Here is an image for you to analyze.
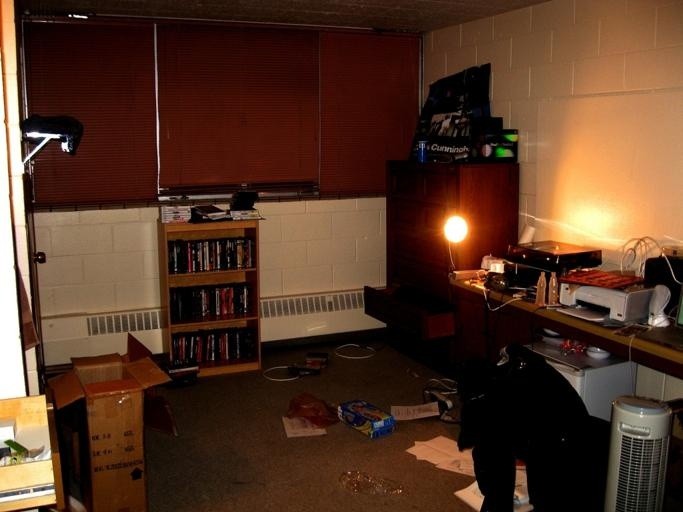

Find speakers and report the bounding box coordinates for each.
[650,286,672,328]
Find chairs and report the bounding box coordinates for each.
[468,345,613,511]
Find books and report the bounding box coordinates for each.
[160,199,259,386]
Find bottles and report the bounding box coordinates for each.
[548,272,556,305]
[535,271,546,305]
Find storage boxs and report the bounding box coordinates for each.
[44,331,170,512]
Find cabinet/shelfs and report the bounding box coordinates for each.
[156,216,262,378]
[383,159,519,364]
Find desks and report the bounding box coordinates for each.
[448,267,683,381]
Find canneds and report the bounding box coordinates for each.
[418,140,428,163]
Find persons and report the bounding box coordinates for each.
[426,111,469,138]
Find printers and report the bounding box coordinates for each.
[557,281,650,324]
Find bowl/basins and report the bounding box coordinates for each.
[585,348,609,359]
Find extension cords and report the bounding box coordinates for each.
[427,390,455,410]
